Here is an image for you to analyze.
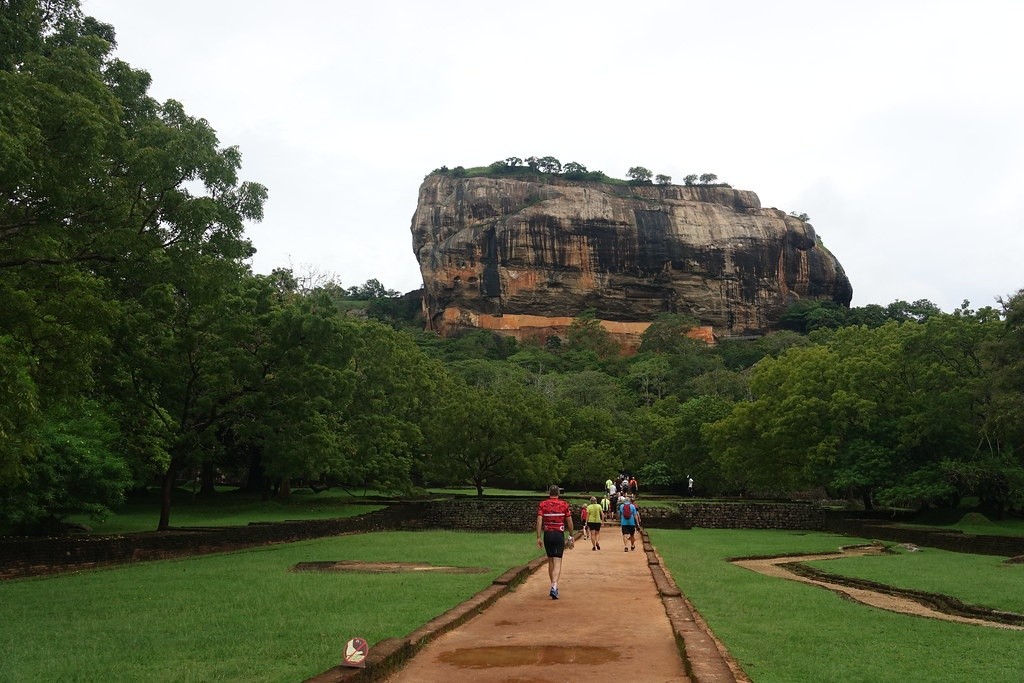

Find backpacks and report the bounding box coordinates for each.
[623,503,632,519]
[631,480,636,491]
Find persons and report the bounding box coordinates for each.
[535,474,694,601]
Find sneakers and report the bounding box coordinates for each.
[550,589,559,599]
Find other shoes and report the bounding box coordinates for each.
[624,546,628,551]
[584,536,589,540]
[592,543,600,551]
[631,546,635,550]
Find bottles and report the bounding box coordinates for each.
[568,535,572,550]
